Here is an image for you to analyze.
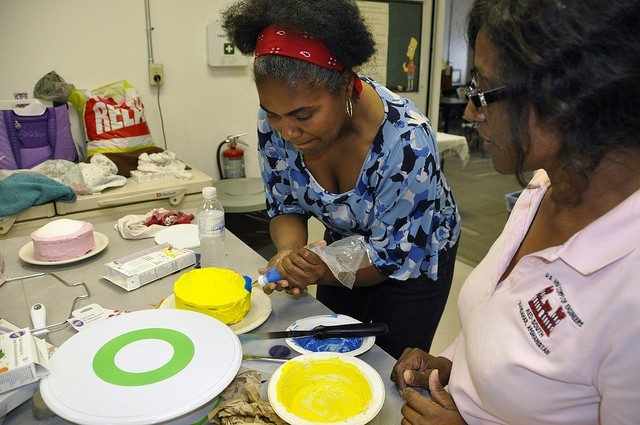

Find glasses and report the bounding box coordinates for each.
[465,69,530,109]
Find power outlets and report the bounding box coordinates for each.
[148,62,163,87]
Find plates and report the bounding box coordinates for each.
[154,224,203,248]
[268,352,385,425]
[18,231,109,266]
[159,285,273,334]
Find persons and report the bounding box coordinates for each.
[219,1,463,361]
[390,0,640,425]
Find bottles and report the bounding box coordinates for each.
[198,186,227,268]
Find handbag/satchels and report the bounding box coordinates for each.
[70,79,155,175]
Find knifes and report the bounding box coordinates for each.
[239,324,386,340]
[286,314,377,357]
[242,355,292,362]
[31,304,53,346]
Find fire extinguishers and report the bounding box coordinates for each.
[217,132,249,180]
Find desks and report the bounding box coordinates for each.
[432,129,469,173]
[440,97,469,133]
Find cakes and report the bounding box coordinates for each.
[27,213,98,259]
[175,264,254,324]
